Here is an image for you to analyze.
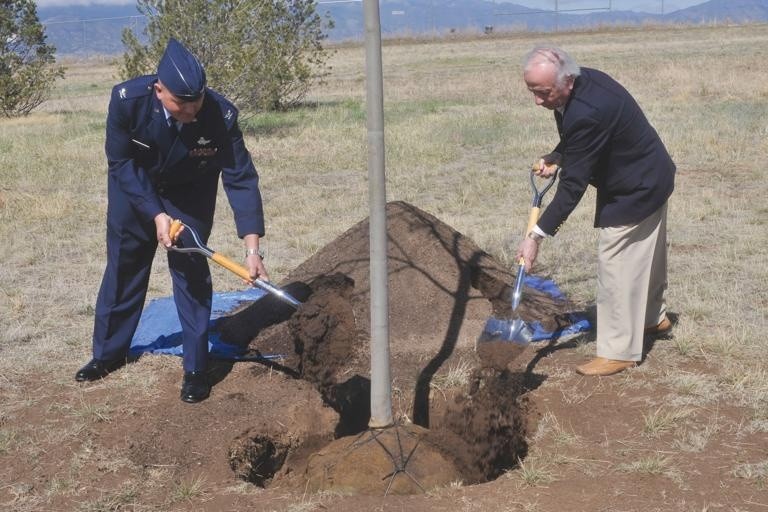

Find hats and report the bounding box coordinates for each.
[156,35,207,103]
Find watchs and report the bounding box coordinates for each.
[528,232,543,245]
[244,248,265,261]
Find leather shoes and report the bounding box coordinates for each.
[179,370,212,404]
[646,315,673,337]
[576,356,636,377]
[74,357,124,382]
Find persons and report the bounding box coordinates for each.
[75,37,270,403]
[516,44,676,377]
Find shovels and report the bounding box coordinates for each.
[476,162,558,365]
[164,219,357,352]
